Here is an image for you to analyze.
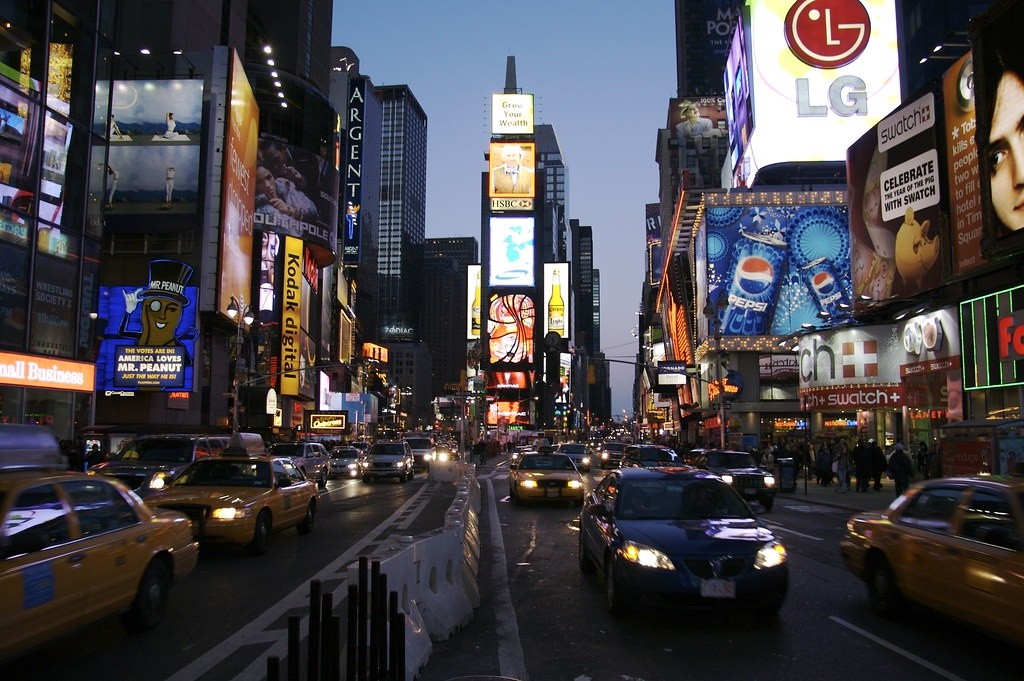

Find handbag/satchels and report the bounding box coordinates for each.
[831,462,838,473]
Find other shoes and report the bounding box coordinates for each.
[842,490,847,494]
[855,488,860,492]
[861,489,868,493]
[834,486,838,492]
[817,481,819,484]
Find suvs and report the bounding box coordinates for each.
[362,439,415,483]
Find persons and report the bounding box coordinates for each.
[254,136,320,237]
[504,438,527,454]
[468,438,487,466]
[57,437,129,468]
[96,102,184,208]
[317,438,354,451]
[676,99,712,139]
[654,439,742,458]
[492,145,534,194]
[672,481,723,521]
[972,30,1024,263]
[760,432,941,496]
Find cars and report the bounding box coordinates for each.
[683,448,775,509]
[328,446,362,479]
[579,467,789,624]
[85,435,231,499]
[142,433,320,557]
[403,431,435,470]
[618,444,682,470]
[266,442,331,488]
[555,443,593,472]
[509,438,585,507]
[0,423,198,658]
[601,442,626,469]
[841,476,1024,645]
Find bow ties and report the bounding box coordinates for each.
[505,169,518,175]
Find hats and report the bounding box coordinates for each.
[679,99,698,119]
[868,439,874,443]
[895,443,903,450]
